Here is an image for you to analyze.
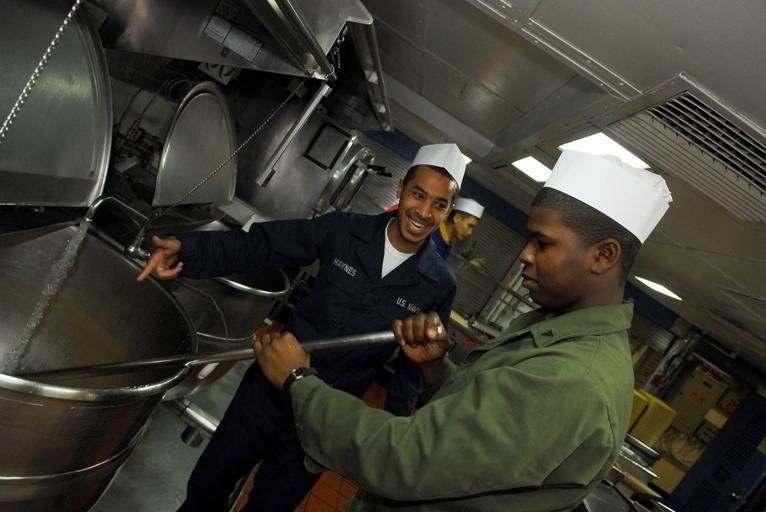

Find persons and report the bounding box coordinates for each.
[133,164,458,510]
[433,208,480,266]
[253,187,643,510]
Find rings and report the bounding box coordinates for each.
[156,266,167,271]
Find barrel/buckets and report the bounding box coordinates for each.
[151,79,291,392]
[0,1,200,512]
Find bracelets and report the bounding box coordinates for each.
[279,366,316,391]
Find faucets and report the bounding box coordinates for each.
[83,195,152,263]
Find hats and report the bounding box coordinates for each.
[543,150,674,245]
[409,143,466,191]
[452,198,485,219]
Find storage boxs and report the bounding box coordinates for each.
[627,387,675,449]
